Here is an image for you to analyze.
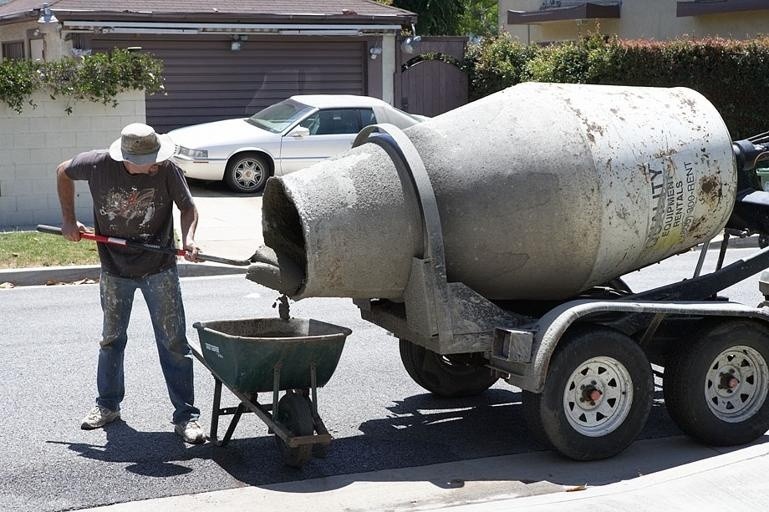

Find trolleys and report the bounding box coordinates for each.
[186,317,352,468]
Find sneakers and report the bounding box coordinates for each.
[173,418,206,444]
[80,401,121,429]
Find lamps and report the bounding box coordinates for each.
[38,6,59,25]
[401,24,421,55]
[370,37,381,59]
[230,34,247,50]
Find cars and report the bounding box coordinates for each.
[165,93,429,193]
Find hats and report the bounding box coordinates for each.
[108,121,175,164]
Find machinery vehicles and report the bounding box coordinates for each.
[263,81,769,461]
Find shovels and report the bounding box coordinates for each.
[36,224,305,297]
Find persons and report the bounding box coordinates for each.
[53,122,211,444]
